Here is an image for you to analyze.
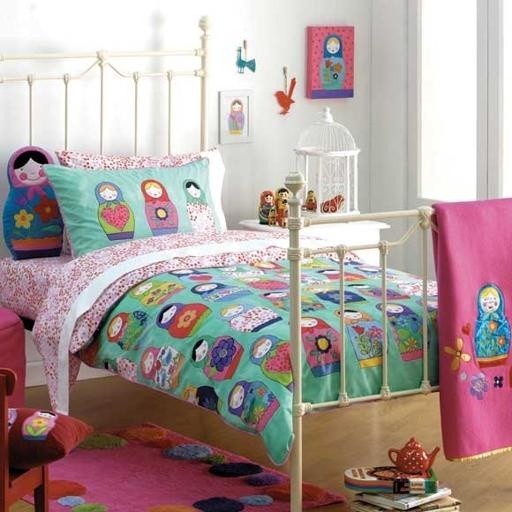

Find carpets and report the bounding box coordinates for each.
[21,420,347,512]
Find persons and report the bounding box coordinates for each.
[307,190,317,211]
[259,186,290,228]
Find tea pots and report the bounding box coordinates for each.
[387,436,441,475]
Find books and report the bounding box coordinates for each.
[350,485,460,511]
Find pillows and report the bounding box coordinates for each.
[42,145,226,261]
[8,408,94,470]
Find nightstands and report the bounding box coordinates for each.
[238,218,391,268]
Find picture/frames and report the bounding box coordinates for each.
[218,90,253,145]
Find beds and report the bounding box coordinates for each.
[0,16,512,511]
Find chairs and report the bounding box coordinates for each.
[0,367,50,512]
